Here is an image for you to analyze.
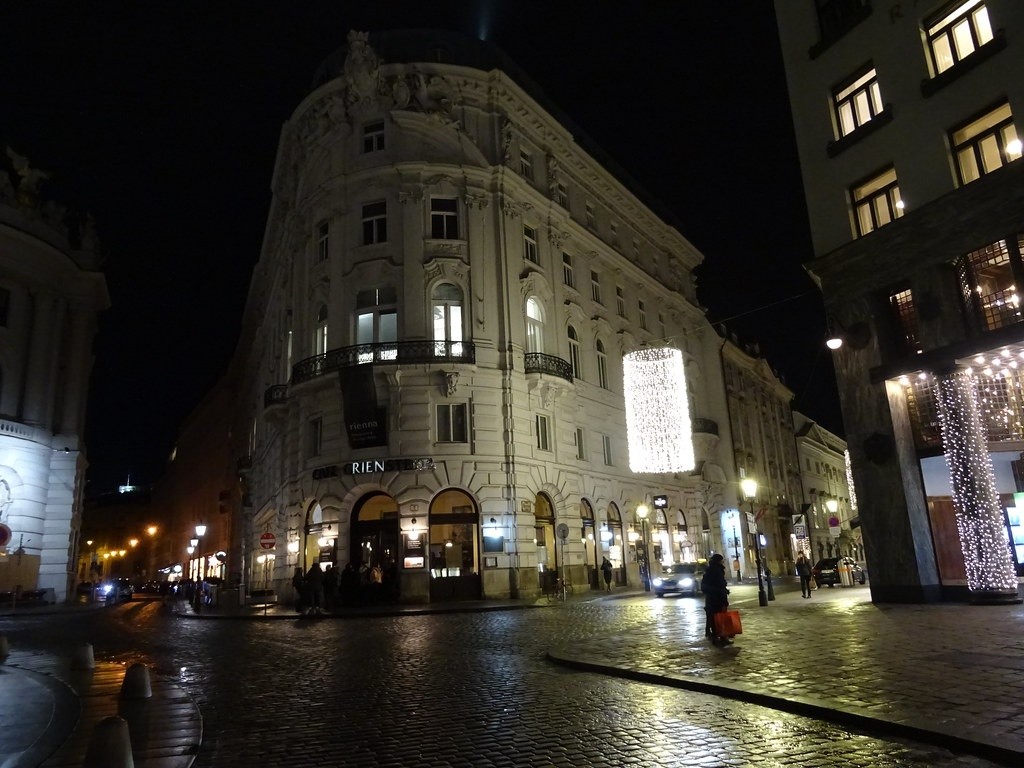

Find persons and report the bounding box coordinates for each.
[170,576,210,614]
[601,559,613,592]
[292,558,383,616]
[344,30,386,104]
[795,550,813,598]
[0,156,99,270]
[702,554,735,647]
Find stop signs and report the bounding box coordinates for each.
[260,533,276,549]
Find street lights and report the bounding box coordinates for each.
[743,479,768,607]
[188,535,202,612]
[826,496,848,588]
[194,519,206,612]
[637,503,651,591]
[824,313,871,350]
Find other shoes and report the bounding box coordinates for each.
[712,639,734,644]
[802,595,806,598]
[607,589,611,592]
[806,596,811,599]
[308,606,321,615]
[705,632,715,637]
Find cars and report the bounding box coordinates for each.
[110,578,133,599]
[653,563,706,597]
[812,557,865,585]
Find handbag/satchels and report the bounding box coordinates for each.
[714,611,742,637]
[809,576,818,591]
[607,566,612,571]
